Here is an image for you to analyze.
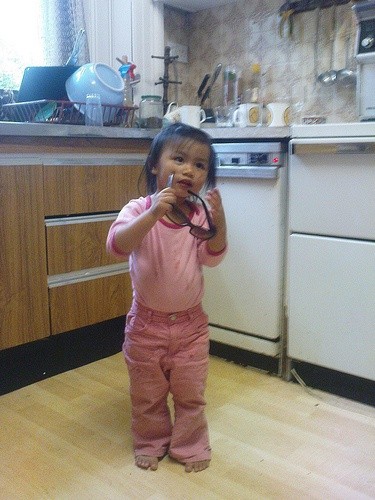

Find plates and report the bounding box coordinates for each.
[34,102,58,123]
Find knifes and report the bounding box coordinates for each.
[192,63,222,108]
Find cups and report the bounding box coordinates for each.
[266,102,290,127]
[181,105,206,129]
[232,103,263,127]
[212,105,236,127]
[85,93,103,126]
[164,101,182,124]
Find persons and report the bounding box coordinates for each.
[105,122,229,472]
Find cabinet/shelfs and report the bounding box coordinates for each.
[0,135,163,396]
[284,137,375,383]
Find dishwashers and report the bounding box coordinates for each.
[187,138,289,378]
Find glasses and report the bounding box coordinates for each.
[165,173,217,240]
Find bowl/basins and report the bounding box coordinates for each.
[16,66,80,100]
[65,63,124,123]
